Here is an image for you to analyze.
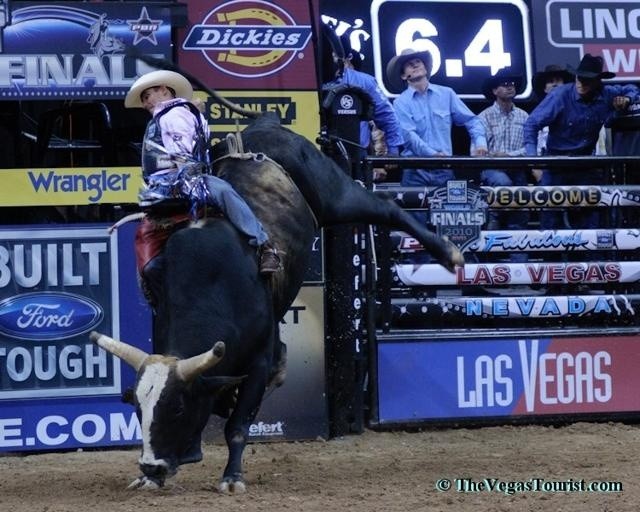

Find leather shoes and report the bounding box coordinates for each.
[260,241,284,273]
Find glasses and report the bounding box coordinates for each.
[500,81,517,86]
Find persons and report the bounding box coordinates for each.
[328,48,640,296]
[124,70,281,303]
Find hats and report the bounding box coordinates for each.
[124,70,193,109]
[386,48,433,90]
[484,53,616,88]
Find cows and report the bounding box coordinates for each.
[87,50,467,495]
